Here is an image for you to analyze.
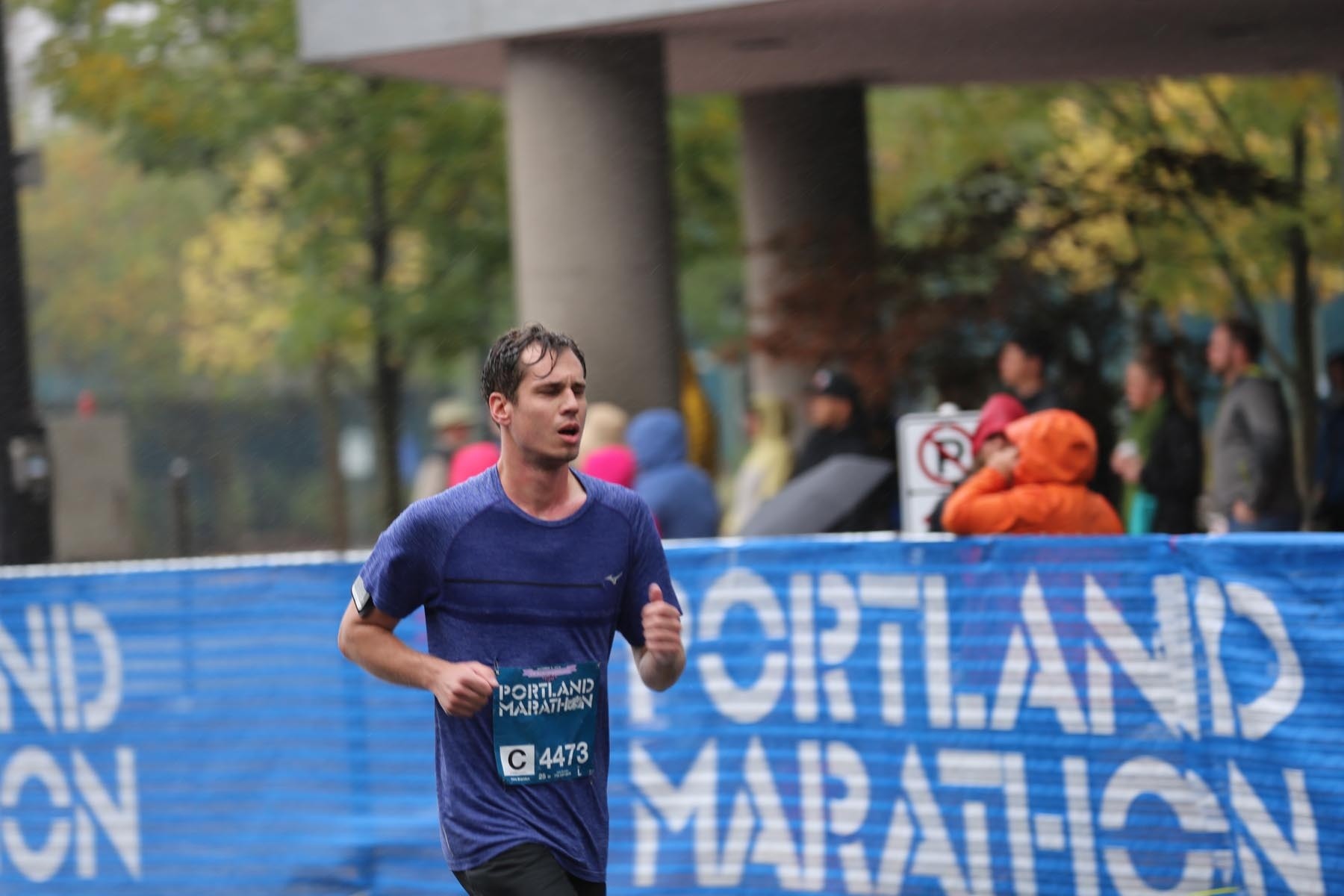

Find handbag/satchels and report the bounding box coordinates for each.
[1126,490,1158,534]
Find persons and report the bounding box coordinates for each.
[335,326,688,896]
[410,323,1344,535]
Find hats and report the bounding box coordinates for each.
[804,368,857,400]
